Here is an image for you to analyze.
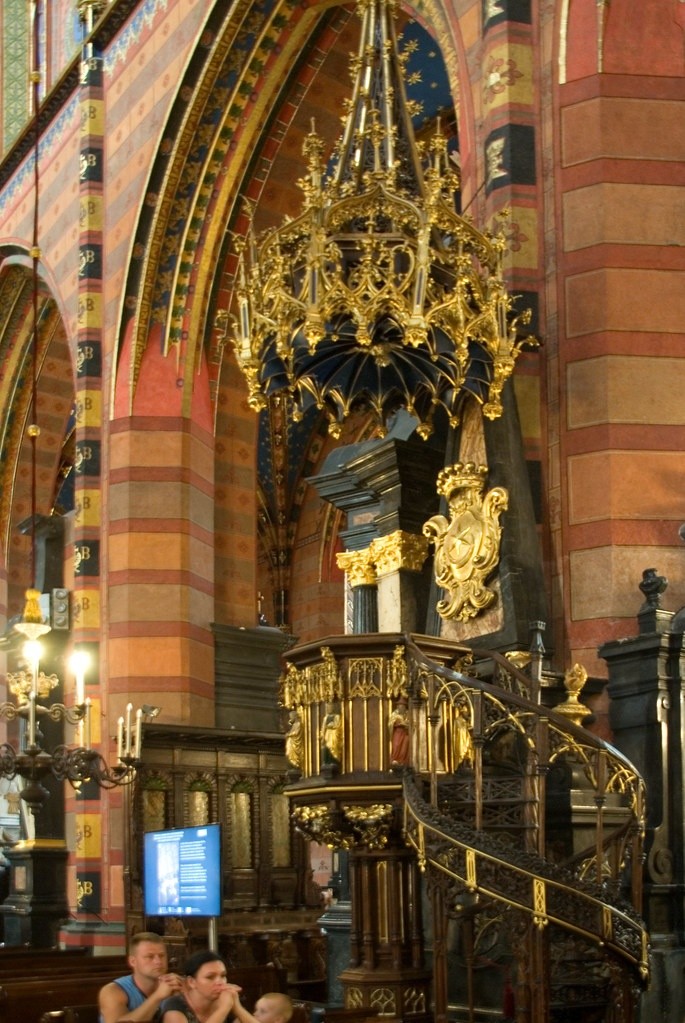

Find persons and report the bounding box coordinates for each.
[159,951,240,1023]
[231,988,292,1022]
[99,932,183,1023]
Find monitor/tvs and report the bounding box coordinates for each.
[143,822,223,917]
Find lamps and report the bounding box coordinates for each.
[0,1,148,816]
